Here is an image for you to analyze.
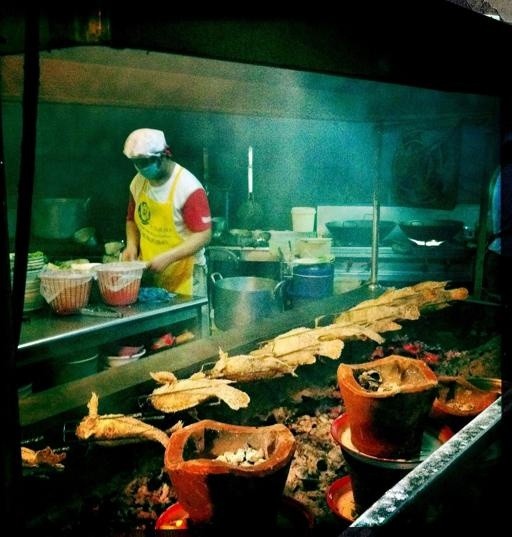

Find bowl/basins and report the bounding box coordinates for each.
[101,345,146,369]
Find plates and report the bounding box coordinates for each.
[10,252,45,318]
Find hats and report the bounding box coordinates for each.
[122,128,169,160]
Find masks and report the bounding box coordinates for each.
[133,162,162,181]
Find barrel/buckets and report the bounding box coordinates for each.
[45,350,98,383]
[103,348,145,372]
[291,207,316,232]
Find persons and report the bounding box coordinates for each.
[120,128,213,340]
[485,138,511,293]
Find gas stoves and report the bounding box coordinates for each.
[330,239,468,265]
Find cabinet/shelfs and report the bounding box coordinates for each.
[16,294,210,388]
[334,244,450,282]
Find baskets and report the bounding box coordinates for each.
[37,269,97,315]
[95,262,146,306]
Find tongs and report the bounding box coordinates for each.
[80,306,123,318]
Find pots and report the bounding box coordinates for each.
[324,213,398,244]
[399,214,464,242]
[30,193,93,240]
[209,272,287,332]
